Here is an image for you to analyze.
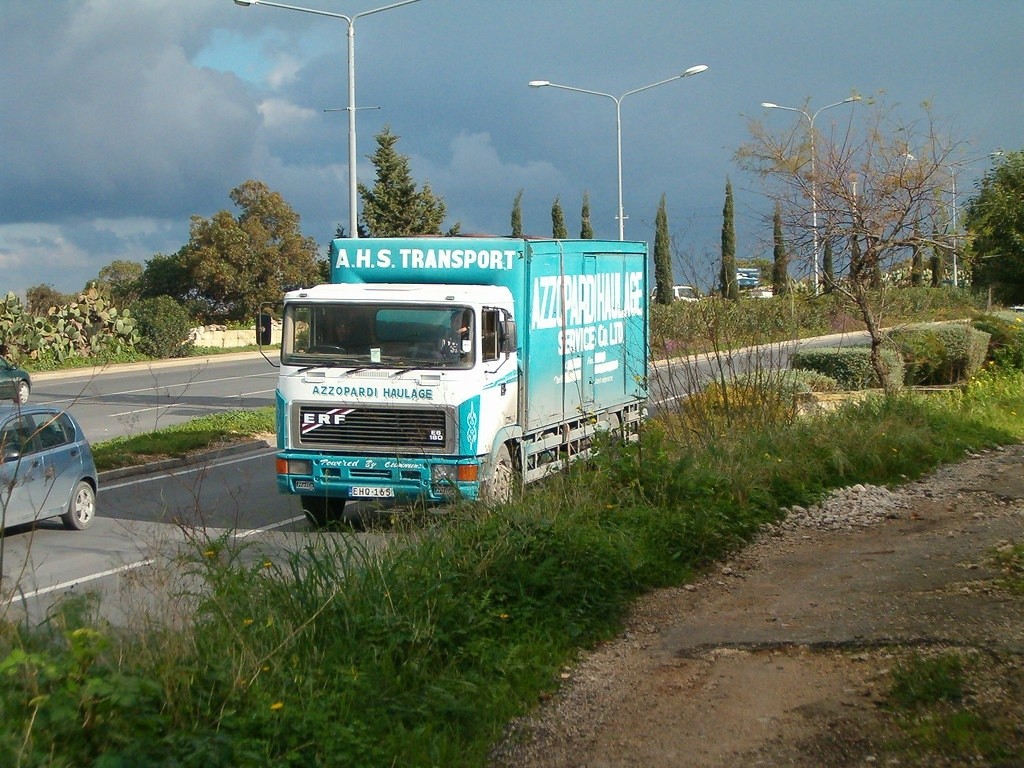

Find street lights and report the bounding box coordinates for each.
[527,64,710,240]
[904,152,1005,287]
[761,94,861,294]
[235,1,423,237]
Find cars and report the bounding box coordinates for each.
[0,407,98,530]
[0,356,32,405]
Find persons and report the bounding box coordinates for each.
[432,312,469,358]
[331,324,367,354]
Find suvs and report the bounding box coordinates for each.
[650,285,703,306]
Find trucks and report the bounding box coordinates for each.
[254,234,650,532]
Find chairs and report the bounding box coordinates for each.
[2,422,59,453]
